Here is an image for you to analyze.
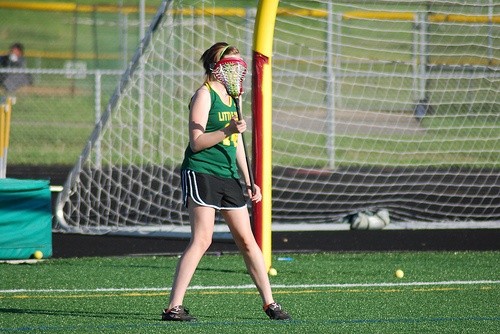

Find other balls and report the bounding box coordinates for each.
[35,251,43,259]
[269,268,277,276]
[395,270,404,279]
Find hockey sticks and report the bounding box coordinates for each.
[209,57,255,196]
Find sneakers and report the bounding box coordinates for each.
[162,305,197,322]
[262,302,291,320]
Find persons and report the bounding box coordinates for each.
[1,43,33,88]
[162,42,291,322]
